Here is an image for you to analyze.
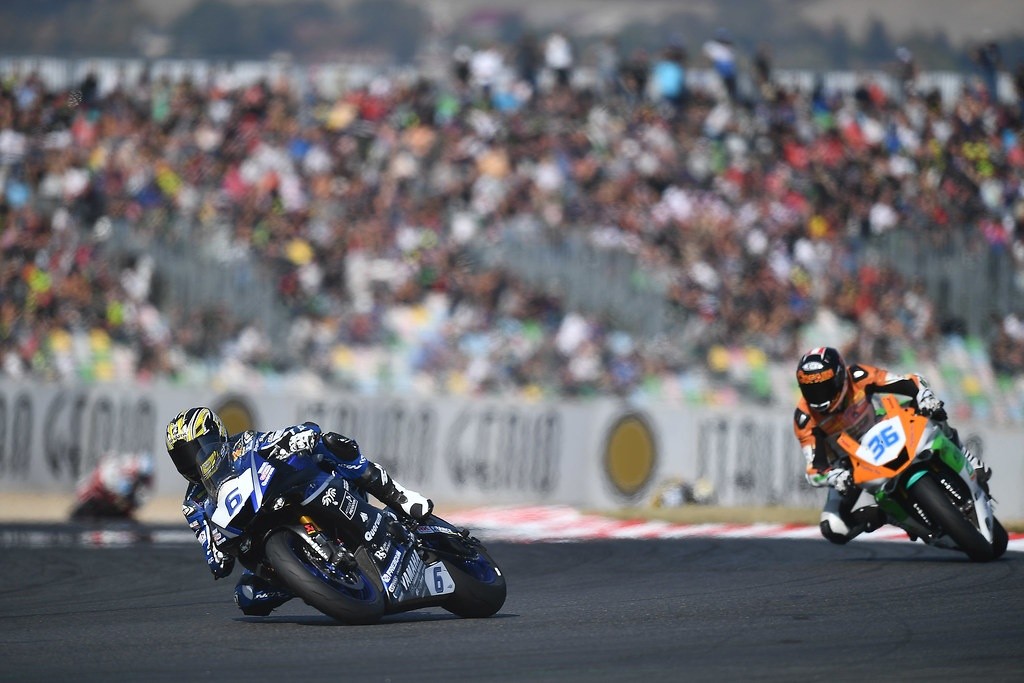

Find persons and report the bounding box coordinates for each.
[95,448,154,514]
[0,32,1024,396]
[165,407,434,616]
[793,347,992,544]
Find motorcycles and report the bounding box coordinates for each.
[208,440,506,626]
[841,394,1009,564]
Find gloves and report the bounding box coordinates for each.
[211,552,235,580]
[917,395,949,421]
[826,467,857,498]
[287,428,320,456]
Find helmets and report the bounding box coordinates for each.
[796,347,849,414]
[164,406,229,483]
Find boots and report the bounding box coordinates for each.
[361,461,434,523]
[946,426,992,484]
